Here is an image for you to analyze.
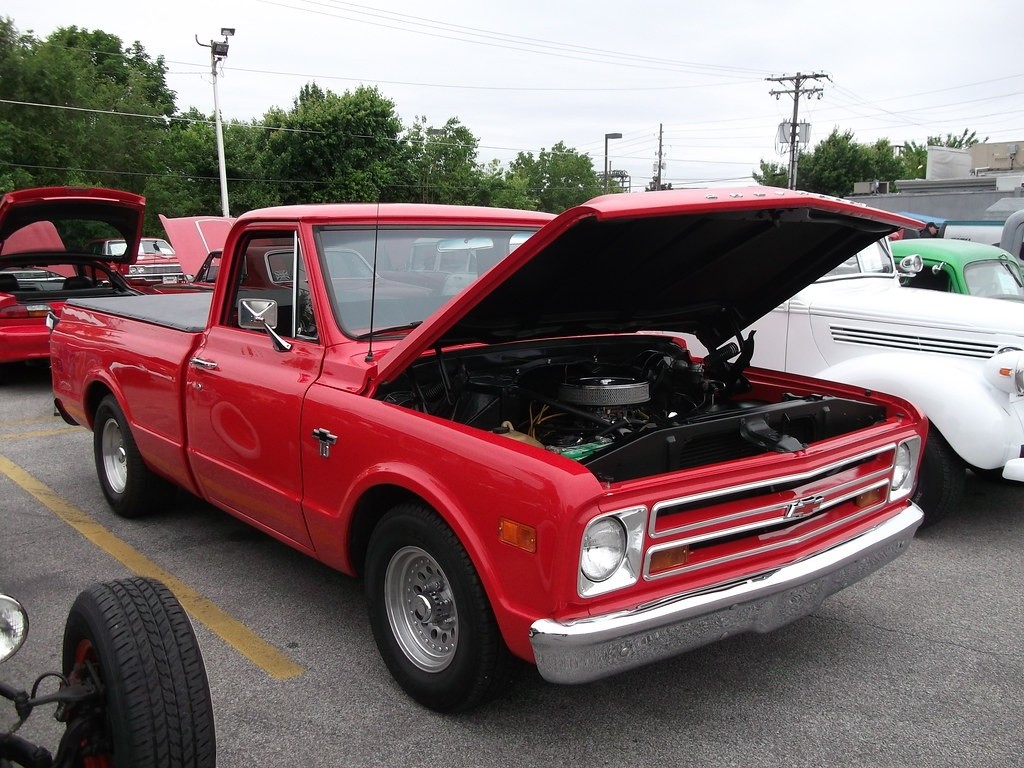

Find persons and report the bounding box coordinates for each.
[920,222,938,238]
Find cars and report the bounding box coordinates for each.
[0,186,1023,524]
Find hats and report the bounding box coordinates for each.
[926,222,939,229]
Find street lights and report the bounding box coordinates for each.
[208,27,236,218]
[603,132,622,193]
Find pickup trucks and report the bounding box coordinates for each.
[46,185,933,711]
